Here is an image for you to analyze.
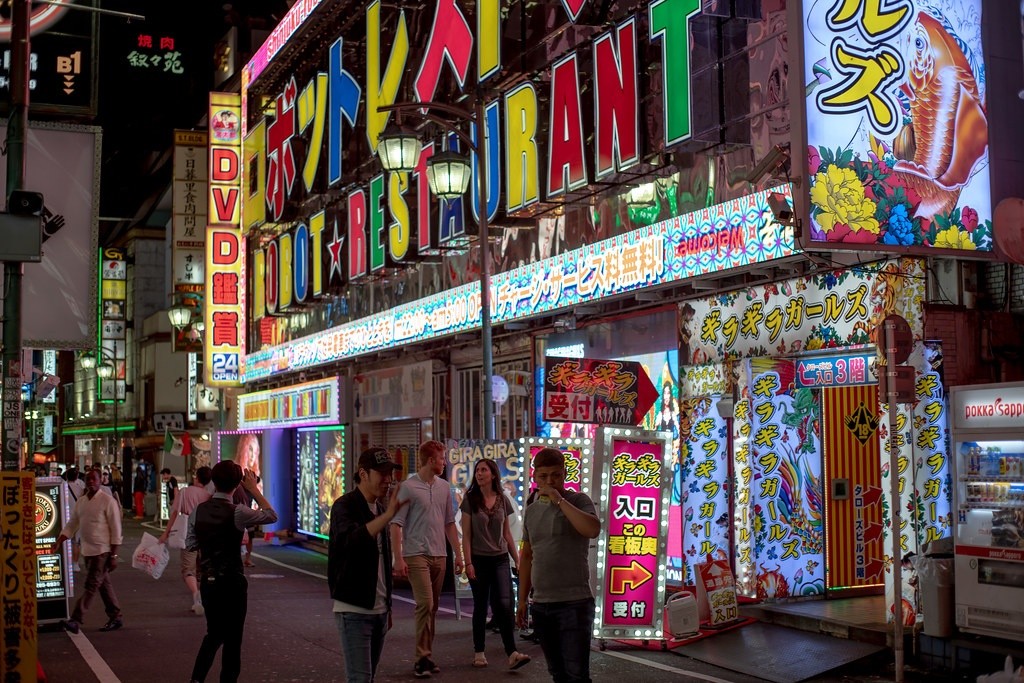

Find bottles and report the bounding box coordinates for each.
[1000,454,1024,477]
[986,449,994,475]
[966,479,1024,502]
[979,447,987,475]
[959,504,1024,525]
[967,446,975,474]
[49,457,57,477]
[973,447,981,474]
[992,450,1000,475]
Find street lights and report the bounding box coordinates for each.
[80,340,117,466]
[376,82,494,444]
[166,291,225,431]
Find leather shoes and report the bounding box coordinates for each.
[99,620,122,631]
[60,620,79,634]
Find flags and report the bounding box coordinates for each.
[163,427,191,456]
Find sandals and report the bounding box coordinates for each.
[472,657,488,667]
[508,653,531,670]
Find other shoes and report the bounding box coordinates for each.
[133,516,144,520]
[492,628,500,633]
[519,632,535,640]
[414,656,432,676]
[195,601,204,615]
[73,565,80,571]
[430,661,440,673]
[243,559,255,567]
[485,619,493,629]
[533,638,540,644]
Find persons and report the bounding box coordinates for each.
[157,467,277,616]
[388,439,462,677]
[515,449,602,683]
[132,467,147,519]
[23,462,124,571]
[459,458,531,669]
[185,459,279,683]
[326,448,410,683]
[655,381,680,436]
[214,111,237,129]
[50,468,124,634]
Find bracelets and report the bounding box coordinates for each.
[558,498,565,506]
[111,555,119,560]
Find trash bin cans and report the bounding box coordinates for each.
[918,550,956,636]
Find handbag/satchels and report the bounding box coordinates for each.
[132,532,169,579]
[167,511,189,549]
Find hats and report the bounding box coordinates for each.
[358,447,403,472]
